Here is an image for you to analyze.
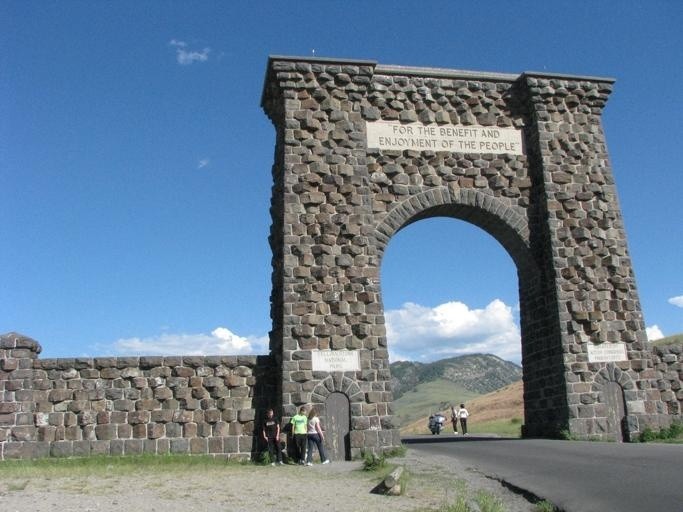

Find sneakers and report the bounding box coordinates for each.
[271,460,284,467]
[322,459,329,465]
[297,459,313,466]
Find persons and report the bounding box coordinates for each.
[450,406,458,434]
[290,407,330,466]
[262,408,284,467]
[458,404,469,436]
